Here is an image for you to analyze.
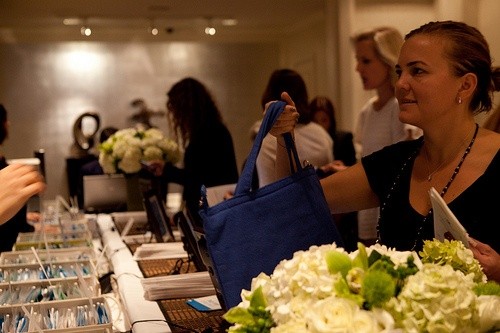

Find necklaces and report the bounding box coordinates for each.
[375,123,479,251]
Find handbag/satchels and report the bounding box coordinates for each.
[197,99,344,313]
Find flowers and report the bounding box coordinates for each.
[222,238,500,333]
[98,126,181,176]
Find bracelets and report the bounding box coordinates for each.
[275,132,295,148]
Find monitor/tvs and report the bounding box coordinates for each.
[171,209,209,275]
[196,234,231,311]
[142,191,176,243]
[84,173,128,213]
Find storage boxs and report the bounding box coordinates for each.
[0,215,113,333]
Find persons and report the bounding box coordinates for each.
[251,68,334,188]
[349,25,423,160]
[0,106,38,252]
[0,157,46,225]
[263,20,500,313]
[156,76,238,273]
[310,95,357,165]
